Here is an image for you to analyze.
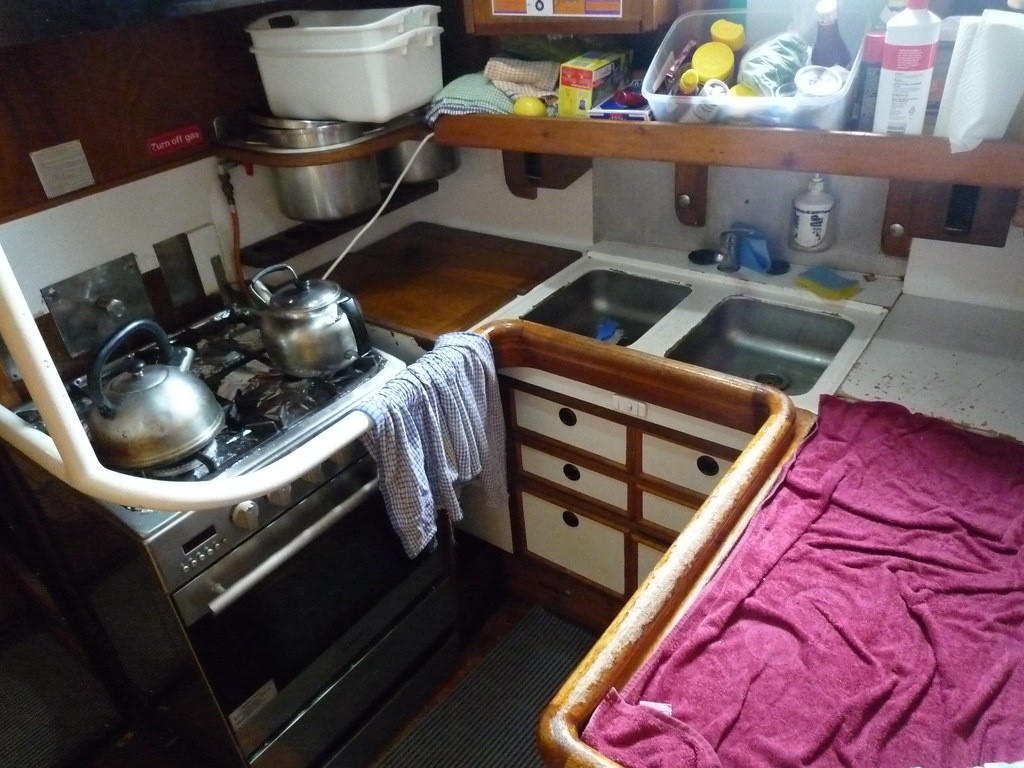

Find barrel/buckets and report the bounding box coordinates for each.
[393,142,463,185]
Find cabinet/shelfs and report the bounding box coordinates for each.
[0,0,285,224]
[463,1,676,36]
[500,367,754,607]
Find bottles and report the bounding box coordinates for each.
[656,71,699,119]
[873,0,941,136]
[790,170,836,254]
[811,0,851,72]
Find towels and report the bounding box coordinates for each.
[578,388,1024,768]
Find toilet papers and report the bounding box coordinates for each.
[929,6,1024,159]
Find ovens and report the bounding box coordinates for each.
[28,459,487,768]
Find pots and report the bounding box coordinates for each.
[254,113,387,223]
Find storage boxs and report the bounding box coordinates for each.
[245,4,446,125]
[640,1,872,132]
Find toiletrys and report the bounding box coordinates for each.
[783,168,834,254]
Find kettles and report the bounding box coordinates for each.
[250,263,372,378]
[85,317,226,470]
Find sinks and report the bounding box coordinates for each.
[521,267,694,352]
[669,287,862,410]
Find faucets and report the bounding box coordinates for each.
[679,222,761,279]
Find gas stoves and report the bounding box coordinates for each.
[0,305,425,590]
